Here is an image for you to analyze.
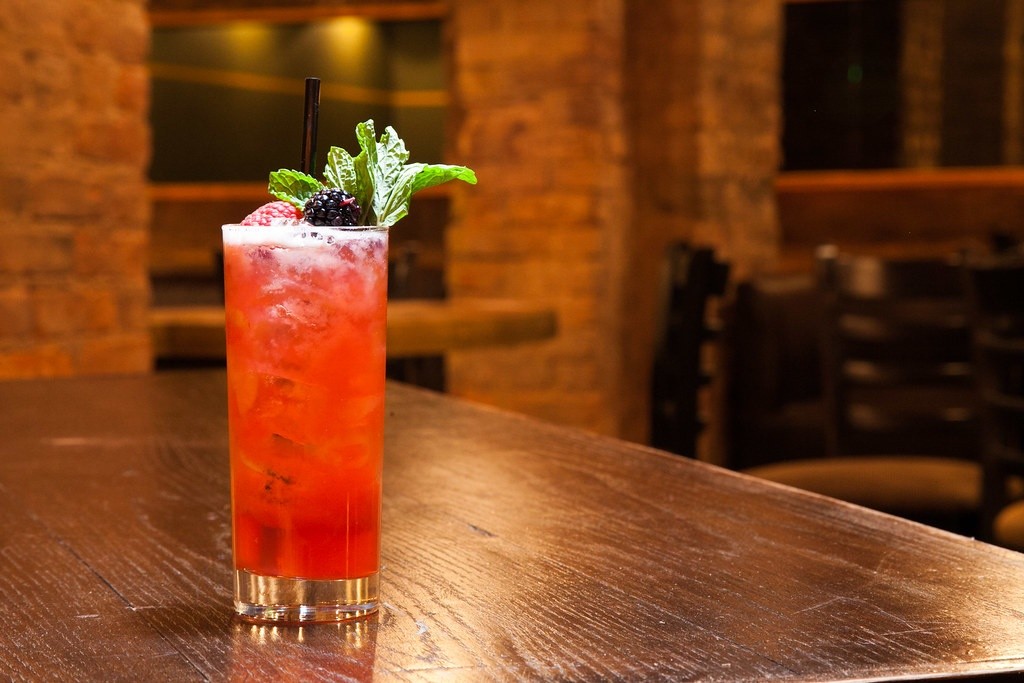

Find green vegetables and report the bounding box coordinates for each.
[269,118,477,226]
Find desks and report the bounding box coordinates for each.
[3,370,1023,683]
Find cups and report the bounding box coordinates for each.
[222,225,390,620]
